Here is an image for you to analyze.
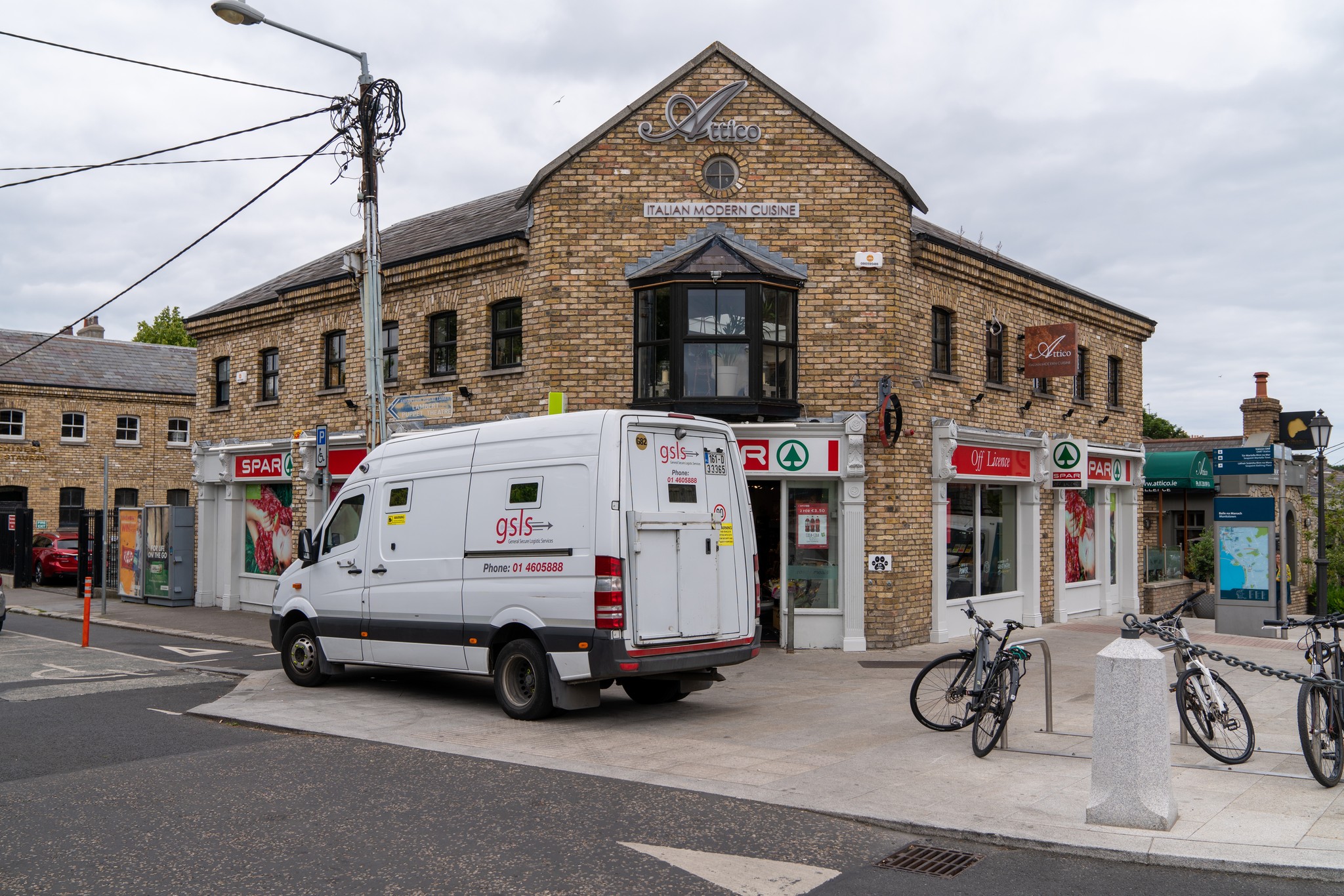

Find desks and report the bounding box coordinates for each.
[744,385,780,398]
[649,383,685,398]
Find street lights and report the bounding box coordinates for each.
[1308,408,1334,629]
[210,0,387,451]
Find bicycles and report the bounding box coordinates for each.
[1132,588,1255,764]
[1260,611,1344,788]
[908,599,1032,758]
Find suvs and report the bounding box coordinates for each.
[32,531,109,587]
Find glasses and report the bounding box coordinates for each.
[1276,557,1280,559]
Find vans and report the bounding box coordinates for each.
[946,514,1003,600]
[267,409,761,722]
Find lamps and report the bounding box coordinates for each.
[1304,518,1311,528]
[711,271,722,279]
[1144,519,1151,528]
[852,376,863,387]
[290,435,361,443]
[1143,508,1170,517]
[911,376,924,389]
[1020,401,1032,411]
[209,443,273,451]
[458,386,472,398]
[971,393,985,408]
[345,400,358,408]
[32,440,40,447]
[728,424,796,429]
[1098,416,1109,425]
[1062,408,1074,418]
[1299,460,1318,477]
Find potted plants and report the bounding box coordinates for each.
[699,296,780,396]
[1182,525,1215,620]
[1289,519,1317,614]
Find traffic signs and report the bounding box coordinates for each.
[386,392,453,421]
[1212,446,1271,462]
[1270,444,1292,462]
[1213,460,1274,475]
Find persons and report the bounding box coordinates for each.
[1065,489,1096,583]
[1275,551,1292,620]
[244,483,293,573]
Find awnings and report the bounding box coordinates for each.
[1142,451,1215,576]
